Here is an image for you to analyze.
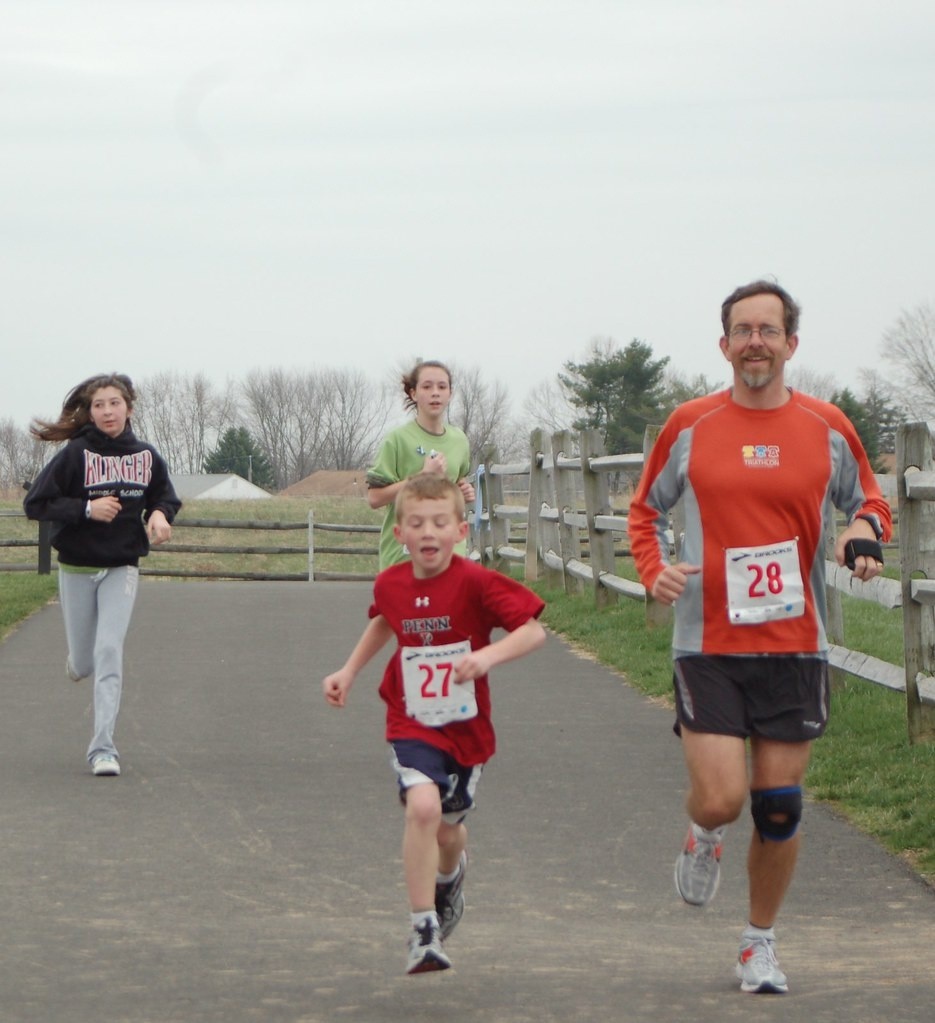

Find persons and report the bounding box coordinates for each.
[23,376,184,778]
[629,280,892,992]
[368,360,474,570]
[320,477,548,975]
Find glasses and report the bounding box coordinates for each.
[727,326,788,339]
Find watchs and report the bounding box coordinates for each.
[85,499,92,521]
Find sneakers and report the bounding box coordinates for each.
[674,820,728,907]
[66,660,81,682]
[437,848,469,939]
[735,936,789,993]
[90,753,121,776]
[405,913,452,975]
